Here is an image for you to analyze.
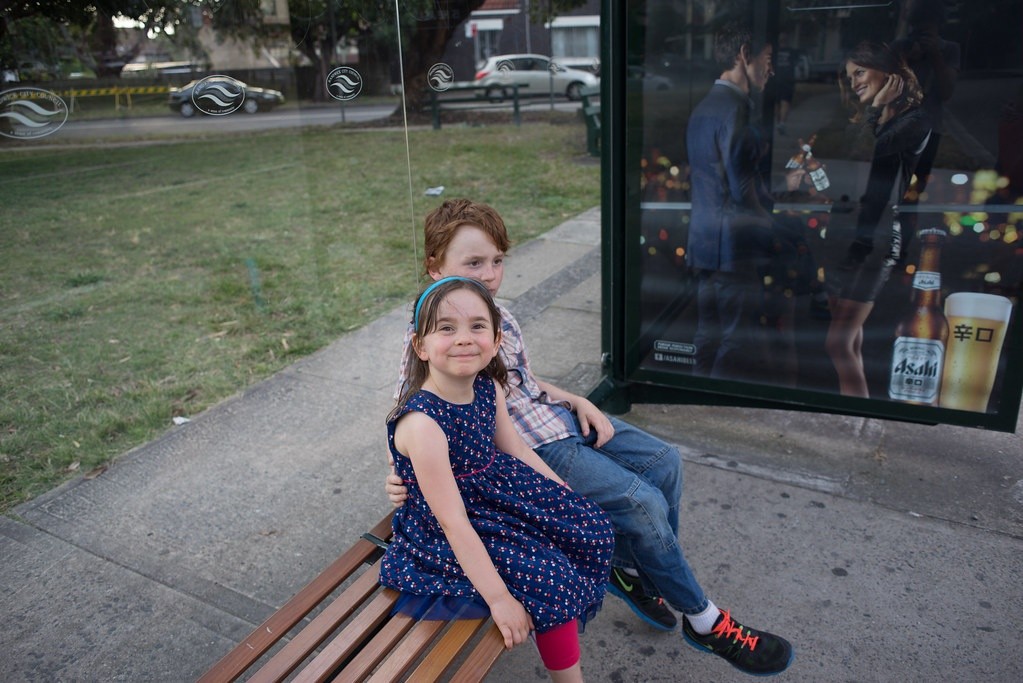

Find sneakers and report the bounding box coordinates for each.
[606,565,677,631]
[681,607,794,677]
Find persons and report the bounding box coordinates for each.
[802,37,938,400]
[681,19,807,381]
[772,32,801,135]
[379,277,615,683]
[386,196,793,676]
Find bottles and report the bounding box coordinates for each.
[797,137,832,193]
[785,134,819,172]
[888,229,949,408]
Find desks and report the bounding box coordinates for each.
[426,83,530,129]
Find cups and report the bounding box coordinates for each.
[939,293,1013,413]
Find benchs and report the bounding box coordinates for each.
[193,507,506,683]
[579,85,601,157]
[420,93,533,113]
